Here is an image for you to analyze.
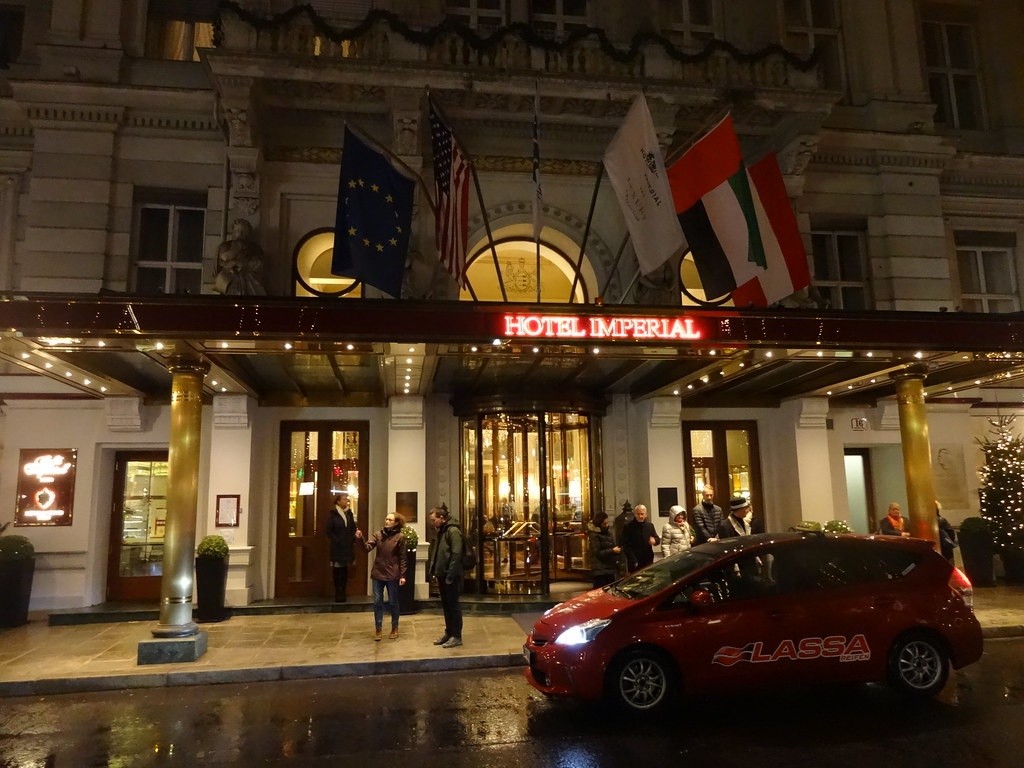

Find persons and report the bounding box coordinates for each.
[934,501,956,567]
[354,512,407,640]
[692,484,725,544]
[879,503,912,536]
[661,505,695,582]
[427,505,468,648]
[585,512,621,589]
[213,217,270,295]
[623,505,660,572]
[718,496,765,599]
[327,495,357,603]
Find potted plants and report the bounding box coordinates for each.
[398,526,420,614]
[195,534,230,623]
[0,535,37,627]
[956,516,994,587]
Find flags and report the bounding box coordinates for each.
[729,148,810,307]
[663,109,768,302]
[603,88,686,276]
[422,100,473,291]
[529,90,544,240]
[330,128,418,302]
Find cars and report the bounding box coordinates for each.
[521,519,986,728]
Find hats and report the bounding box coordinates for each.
[729,496,748,510]
[593,511,608,526]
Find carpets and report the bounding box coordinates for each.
[512,611,544,636]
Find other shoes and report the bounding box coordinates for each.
[389,628,399,639]
[442,634,462,647]
[434,630,451,645]
[374,628,382,641]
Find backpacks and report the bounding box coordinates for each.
[446,525,477,570]
